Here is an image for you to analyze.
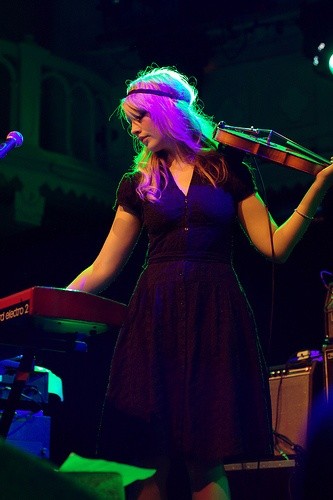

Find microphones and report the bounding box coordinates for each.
[0,130,24,159]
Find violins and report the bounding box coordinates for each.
[213,121,332,176]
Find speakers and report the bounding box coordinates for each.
[267,360,323,459]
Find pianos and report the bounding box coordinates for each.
[0,286,128,343]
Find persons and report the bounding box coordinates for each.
[63,62,332,500]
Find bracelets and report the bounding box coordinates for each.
[294,208,314,221]
